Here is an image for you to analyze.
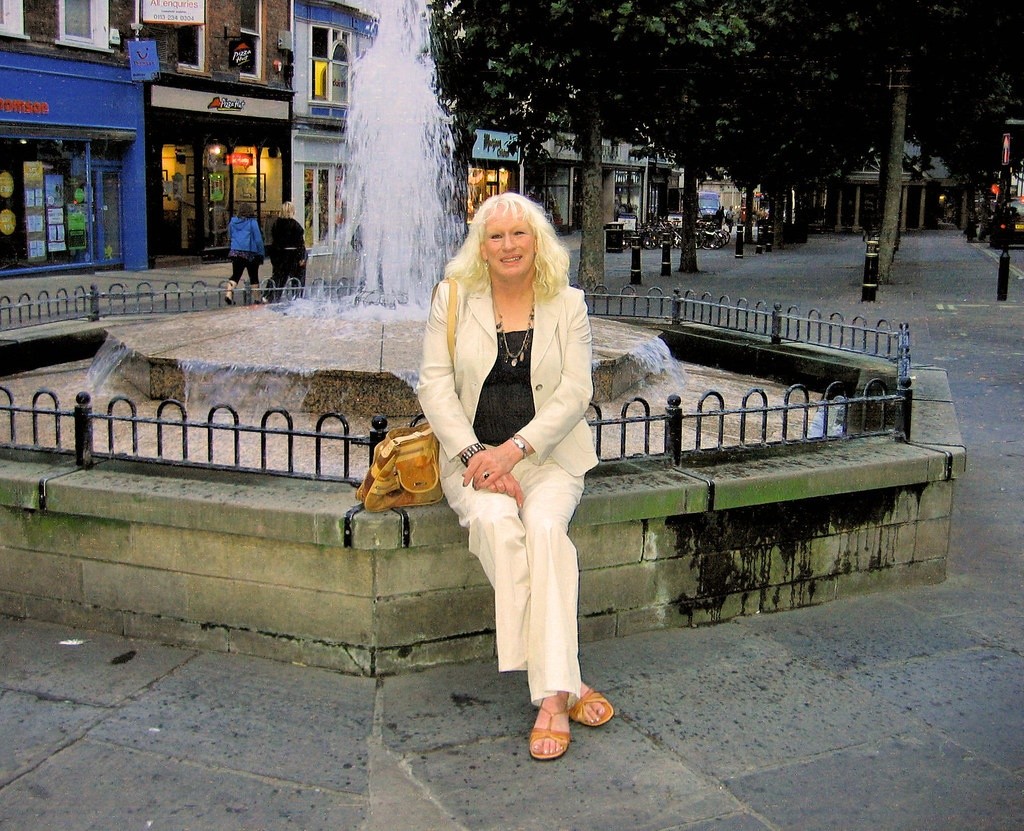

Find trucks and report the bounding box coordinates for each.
[698,190,723,220]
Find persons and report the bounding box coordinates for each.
[225,202,309,305]
[717,204,737,233]
[417,193,615,761]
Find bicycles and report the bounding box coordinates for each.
[621,214,731,250]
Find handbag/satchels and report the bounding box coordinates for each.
[354,422,445,513]
[249,252,264,265]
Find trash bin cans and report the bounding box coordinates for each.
[605,222,625,253]
[741,206,746,224]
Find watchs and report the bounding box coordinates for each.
[461,442,487,467]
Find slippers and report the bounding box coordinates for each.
[224,296,236,305]
[254,300,262,304]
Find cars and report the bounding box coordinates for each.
[989,197,1024,249]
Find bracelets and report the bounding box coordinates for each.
[510,437,527,459]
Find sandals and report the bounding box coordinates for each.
[569,686,614,726]
[530,692,570,760]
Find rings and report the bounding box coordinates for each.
[482,472,490,479]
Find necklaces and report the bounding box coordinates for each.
[494,298,534,367]
